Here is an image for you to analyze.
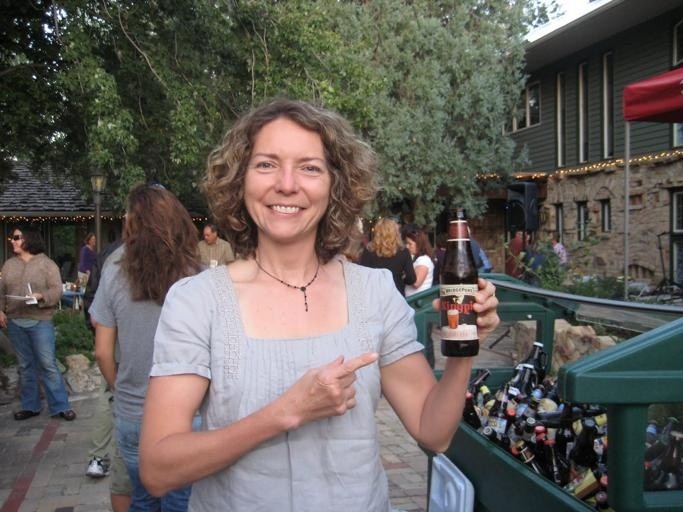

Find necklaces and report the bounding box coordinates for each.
[250,252,319,310]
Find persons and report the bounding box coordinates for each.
[1,226,77,422]
[361,218,417,297]
[110,442,131,512]
[405,229,435,298]
[79,233,124,474]
[548,230,567,268]
[88,185,197,512]
[509,231,531,277]
[75,234,98,310]
[196,223,234,269]
[137,101,501,511]
[466,227,494,275]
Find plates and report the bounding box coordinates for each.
[6,294,34,300]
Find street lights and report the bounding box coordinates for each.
[90,168,107,289]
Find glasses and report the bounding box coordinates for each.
[7,235,21,240]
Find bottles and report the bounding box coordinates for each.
[437,206,480,357]
[459,341,682,512]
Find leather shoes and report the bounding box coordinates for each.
[60,410,77,418]
[16,405,40,420]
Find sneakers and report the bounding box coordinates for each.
[86,459,110,476]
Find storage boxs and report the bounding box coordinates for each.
[405,271,683,512]
